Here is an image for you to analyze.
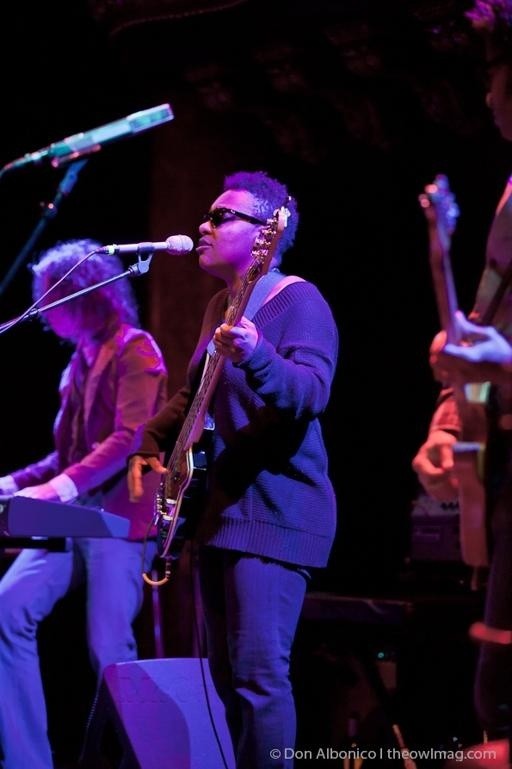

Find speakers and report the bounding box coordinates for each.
[76,657,239,768]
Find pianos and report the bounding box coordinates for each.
[0,495,132,552]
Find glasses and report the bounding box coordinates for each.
[203,208,265,227]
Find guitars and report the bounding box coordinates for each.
[420,177,497,566]
[152,203,293,563]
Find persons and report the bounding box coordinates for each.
[1,237,170,769]
[410,17,512,767]
[126,170,340,768]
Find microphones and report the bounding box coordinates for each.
[97,235,196,257]
